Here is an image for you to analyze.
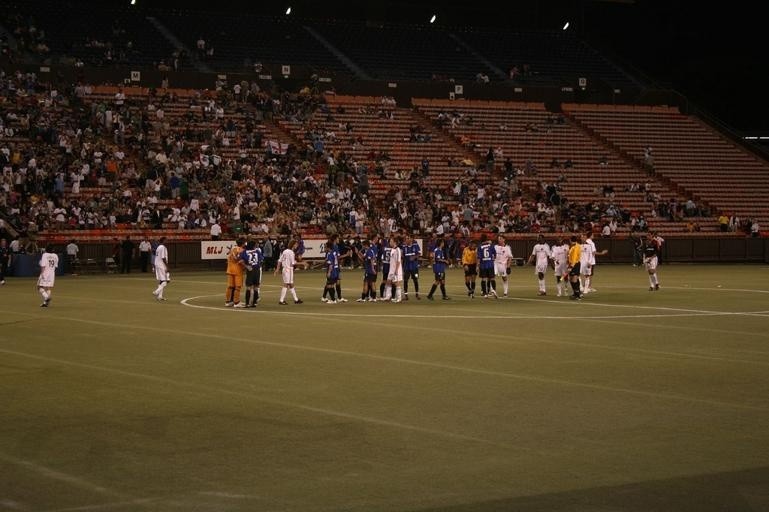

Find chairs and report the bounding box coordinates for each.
[0,2,768,239]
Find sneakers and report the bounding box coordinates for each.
[294,298,303,304]
[538,288,597,300]
[444,295,449,300]
[224,296,261,308]
[427,295,433,301]
[320,297,348,304]
[356,292,421,303]
[279,301,288,305]
[467,290,508,299]
[649,284,660,291]
[151,291,166,301]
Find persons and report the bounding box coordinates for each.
[36,245,58,306]
[312,233,422,304]
[1,0,761,273]
[152,236,170,301]
[427,238,451,300]
[224,237,265,308]
[524,231,608,301]
[2,239,11,284]
[643,230,661,291]
[274,239,309,305]
[462,234,513,300]
